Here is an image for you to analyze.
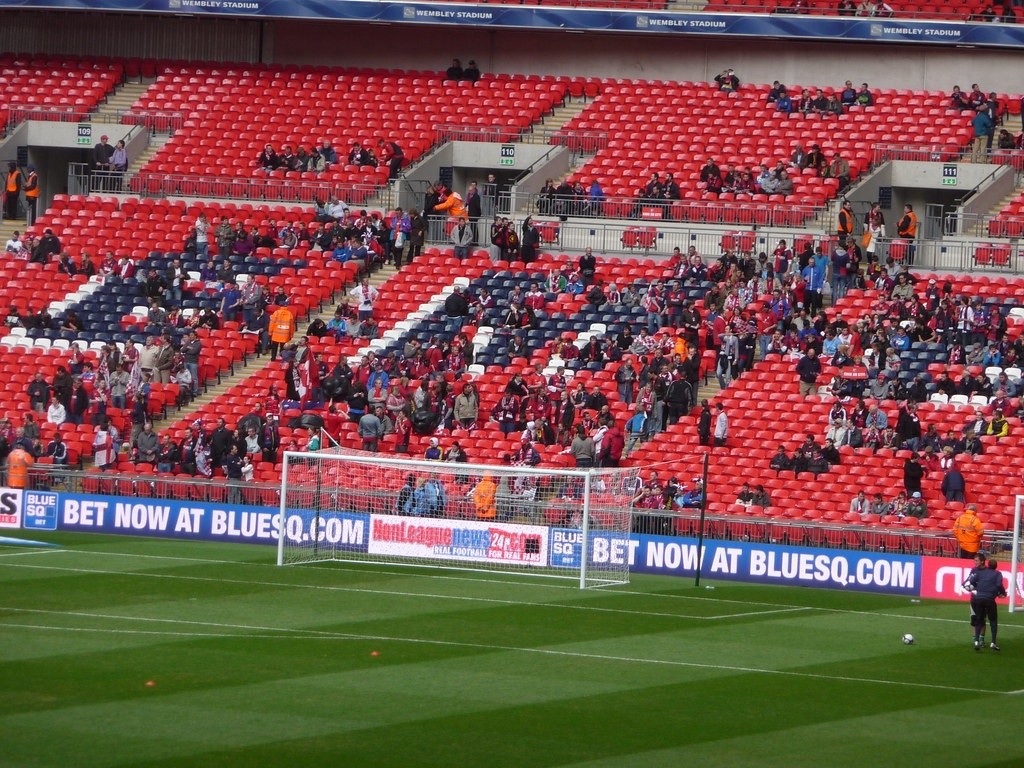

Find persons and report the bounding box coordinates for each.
[951,503,985,558]
[0,58,1024,534]
[999,5,1016,23]
[789,0,810,14]
[960,552,987,648]
[978,4,996,22]
[855,0,876,16]
[838,0,857,16]
[970,559,1008,651]
[871,0,895,17]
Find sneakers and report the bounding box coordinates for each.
[990,643,1000,651]
[980,641,987,647]
[974,641,979,650]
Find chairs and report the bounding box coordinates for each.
[0,46,1024,562]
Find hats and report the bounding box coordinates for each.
[924,445,933,452]
[910,492,922,498]
[943,446,953,452]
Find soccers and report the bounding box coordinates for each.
[902,633,914,645]
